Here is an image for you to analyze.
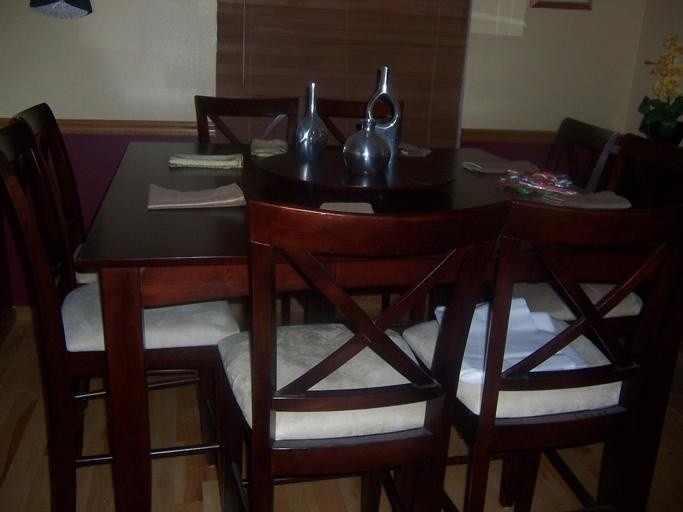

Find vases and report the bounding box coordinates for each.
[650,123,681,144]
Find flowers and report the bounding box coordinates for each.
[638,29,682,139]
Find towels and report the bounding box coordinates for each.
[428,295,591,383]
[540,188,628,212]
[397,138,432,159]
[144,183,246,212]
[169,149,240,173]
[250,137,289,157]
[461,157,538,177]
[315,198,376,215]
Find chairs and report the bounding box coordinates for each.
[303,90,405,321]
[2,110,244,509]
[404,202,682,512]
[192,90,303,318]
[543,112,618,186]
[14,98,158,286]
[512,126,681,342]
[201,192,505,512]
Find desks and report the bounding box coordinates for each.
[72,133,682,511]
